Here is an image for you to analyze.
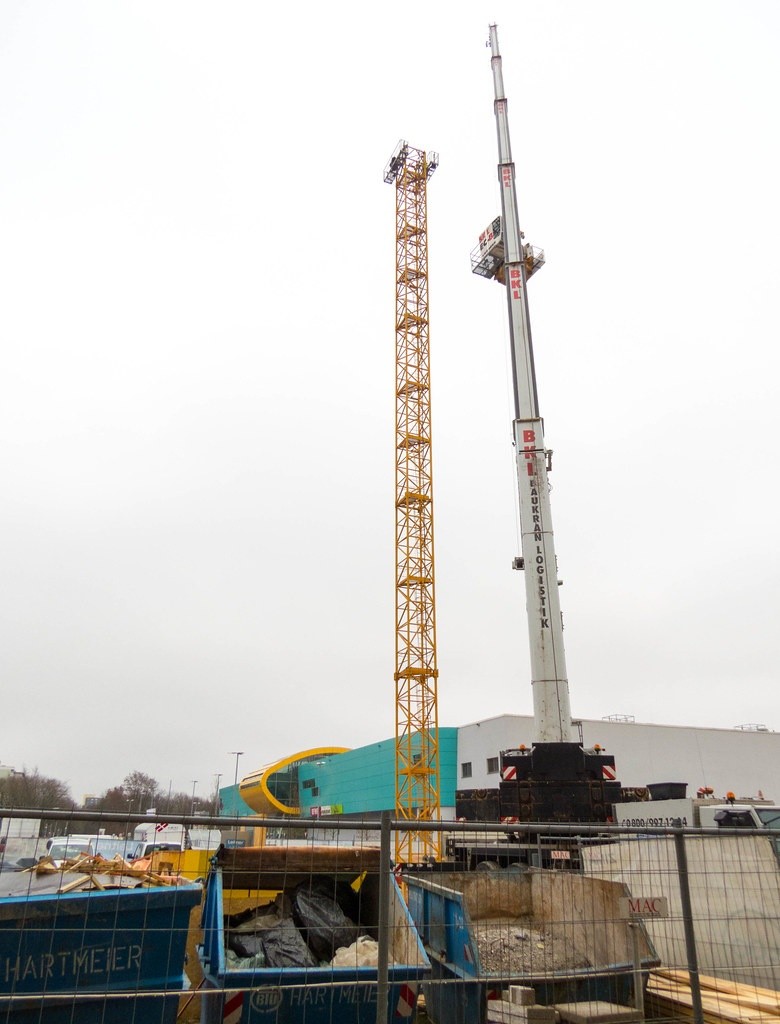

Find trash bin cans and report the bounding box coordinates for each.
[0,872,204,1024]
[400,867,663,1024]
[200,843,433,1024]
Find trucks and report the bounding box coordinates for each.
[612,797,780,859]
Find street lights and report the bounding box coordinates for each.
[228,751,246,832]
[213,774,225,813]
[190,778,199,814]
[124,798,134,839]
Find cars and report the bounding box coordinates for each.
[39,818,223,875]
